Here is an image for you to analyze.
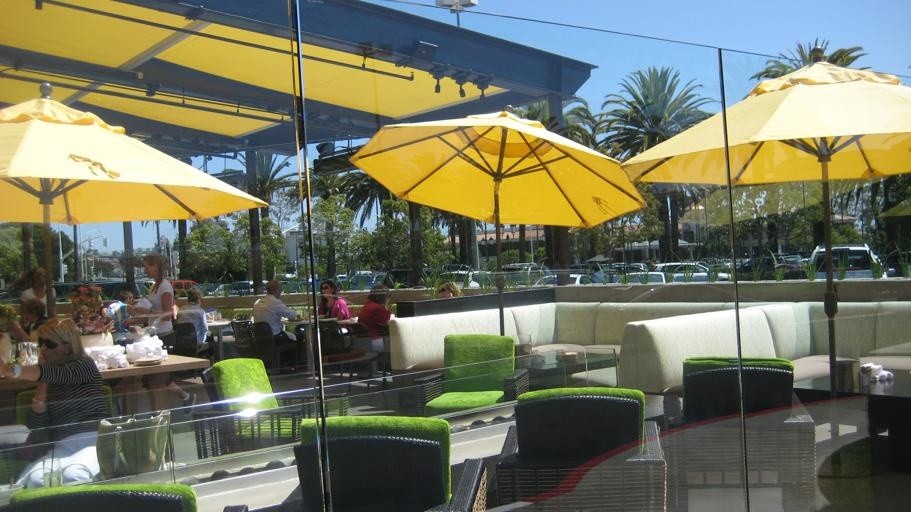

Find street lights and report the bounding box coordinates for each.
[436,0,482,271]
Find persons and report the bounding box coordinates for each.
[0,316,114,445]
[252,280,301,371]
[1,253,196,415]
[313,280,392,374]
[438,280,461,299]
[179,285,216,366]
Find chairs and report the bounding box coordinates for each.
[2,384,117,499]
[193,356,349,462]
[5,274,475,395]
[660,357,821,507]
[280,414,488,512]
[413,335,532,416]
[489,384,670,509]
[1,482,253,512]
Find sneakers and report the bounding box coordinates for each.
[182,391,196,417]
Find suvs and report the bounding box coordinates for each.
[808,243,896,281]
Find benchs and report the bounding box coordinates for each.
[388,302,909,427]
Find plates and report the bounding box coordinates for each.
[134,355,162,365]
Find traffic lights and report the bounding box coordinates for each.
[88,236,94,247]
[63,264,68,276]
[102,237,108,248]
[90,267,96,275]
[173,250,180,265]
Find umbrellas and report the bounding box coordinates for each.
[348,105,649,337]
[0,79,269,321]
[618,46,911,399]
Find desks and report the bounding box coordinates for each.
[793,366,909,471]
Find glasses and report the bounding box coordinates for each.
[37,336,70,350]
[319,285,331,290]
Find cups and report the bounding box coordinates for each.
[18,342,39,366]
[207,313,221,323]
